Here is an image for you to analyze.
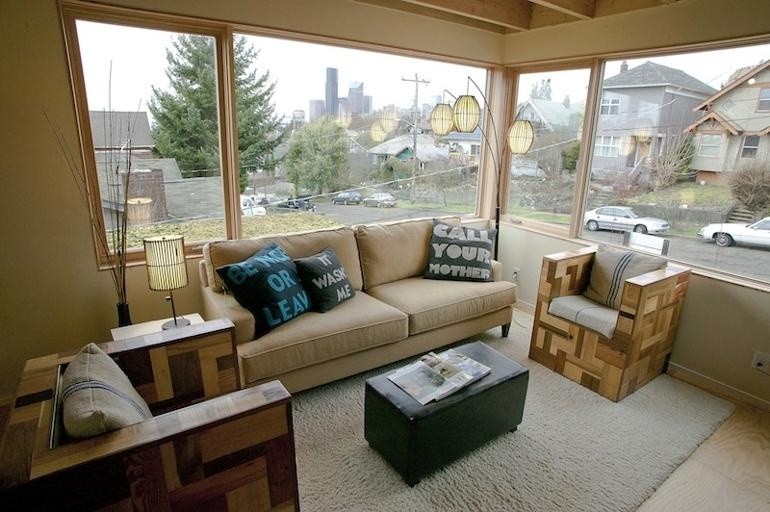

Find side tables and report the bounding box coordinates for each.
[109,312,206,341]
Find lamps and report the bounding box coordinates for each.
[617,133,635,157]
[127,196,152,222]
[370,120,387,144]
[334,107,354,128]
[634,118,653,143]
[381,105,399,134]
[143,233,192,330]
[429,75,535,262]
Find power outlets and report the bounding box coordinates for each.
[511,267,521,283]
[750,349,770,376]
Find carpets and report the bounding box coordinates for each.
[290,305,736,511]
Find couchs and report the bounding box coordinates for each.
[198,215,518,393]
[363,339,530,488]
[527,245,692,403]
[0,318,303,512]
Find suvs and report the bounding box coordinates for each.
[331,191,362,205]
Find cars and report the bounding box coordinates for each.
[583,205,670,235]
[363,193,396,207]
[239,194,266,216]
[698,218,770,249]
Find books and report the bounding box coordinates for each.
[408,351,473,400]
[387,360,455,405]
[434,348,483,387]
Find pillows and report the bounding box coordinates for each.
[61,343,153,443]
[292,247,356,313]
[215,244,313,341]
[582,242,669,311]
[424,218,498,283]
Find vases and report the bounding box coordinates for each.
[115,301,132,327]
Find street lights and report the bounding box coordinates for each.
[394,118,418,206]
